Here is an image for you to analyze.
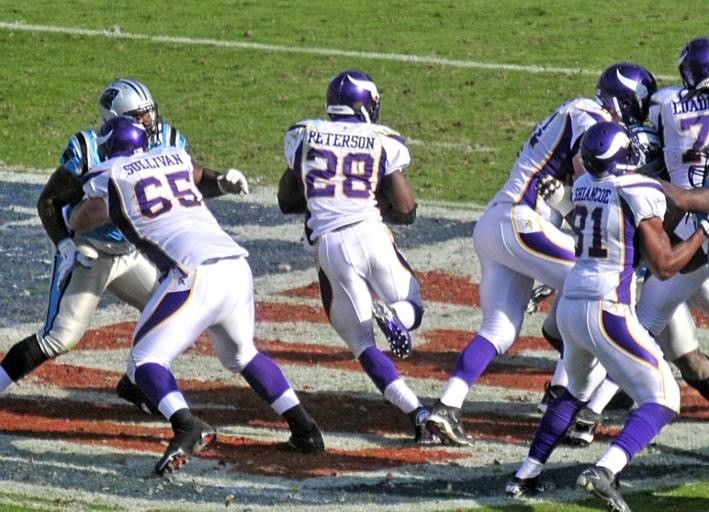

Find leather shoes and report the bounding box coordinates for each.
[534,174,575,217]
[217,169,249,195]
[57,238,92,290]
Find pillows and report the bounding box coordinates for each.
[581,64,663,177]
[326,70,380,123]
[97,79,163,161]
[678,36,709,90]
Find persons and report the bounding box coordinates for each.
[275,68,436,445]
[1,76,252,417]
[503,119,707,512]
[66,112,326,478]
[533,37,706,448]
[426,63,658,450]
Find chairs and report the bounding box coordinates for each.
[503,400,631,512]
[279,423,324,452]
[416,398,471,445]
[371,299,412,359]
[155,417,216,474]
[116,373,163,416]
